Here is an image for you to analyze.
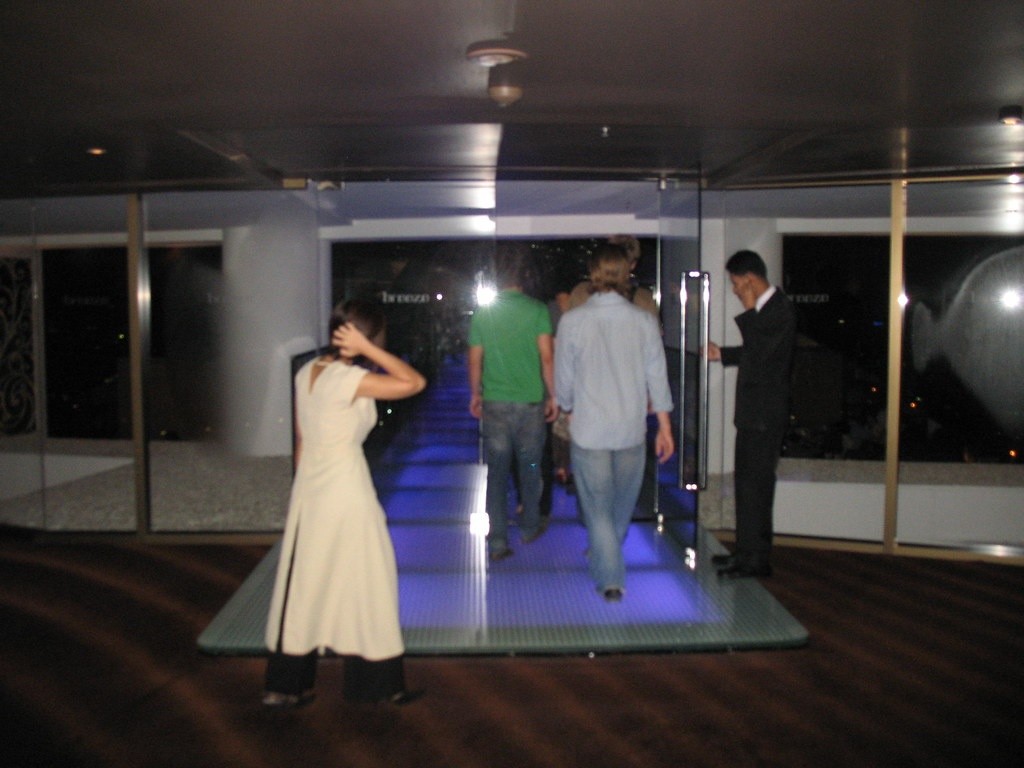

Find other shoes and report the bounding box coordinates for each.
[370,689,425,705]
[712,551,742,564]
[604,584,623,598]
[719,563,771,578]
[523,527,545,542]
[264,695,312,713]
[488,549,513,561]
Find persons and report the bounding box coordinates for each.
[699,249,799,576]
[466,234,679,599]
[261,296,430,711]
